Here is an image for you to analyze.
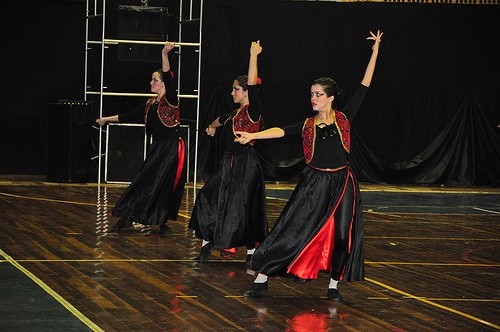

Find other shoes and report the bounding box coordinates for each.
[244,281,268,296]
[114,218,133,230]
[327,288,343,302]
[160,224,172,234]
[246,254,252,269]
[195,242,212,260]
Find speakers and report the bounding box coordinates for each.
[45,103,92,182]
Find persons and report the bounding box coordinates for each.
[234,30,384,303]
[187,39,271,270]
[95,41,186,235]
